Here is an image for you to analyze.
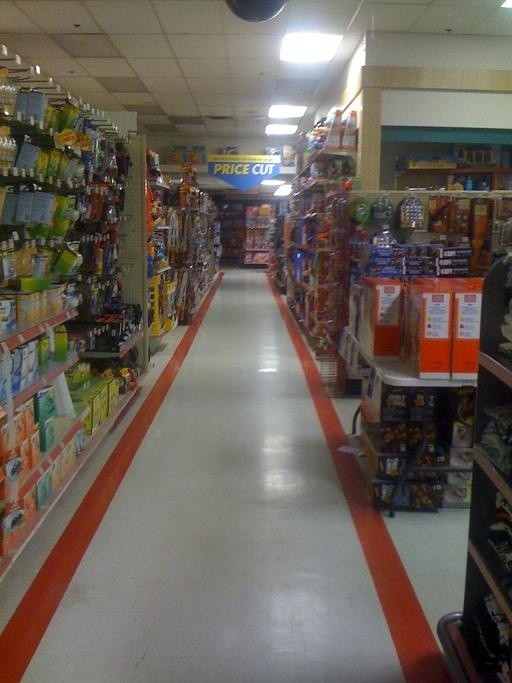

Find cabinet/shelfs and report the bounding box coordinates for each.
[350,192,510,301]
[386,142,507,191]
[148,179,179,338]
[244,215,271,265]
[457,258,512,682]
[345,324,446,516]
[293,125,358,359]
[0,306,141,578]
